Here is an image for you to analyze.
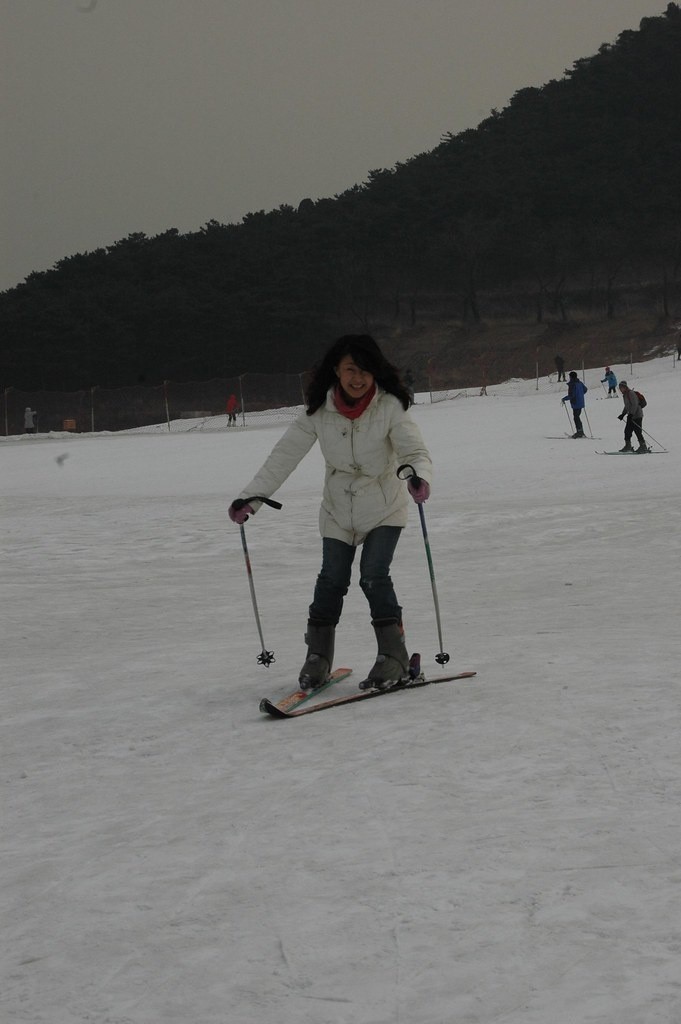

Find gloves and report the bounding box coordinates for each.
[407,478,431,503]
[227,499,252,523]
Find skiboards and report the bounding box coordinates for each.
[595,449,668,456]
[543,432,602,440]
[258,667,477,718]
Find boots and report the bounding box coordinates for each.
[637,442,647,452]
[620,441,631,452]
[572,427,585,438]
[367,619,409,688]
[299,623,335,689]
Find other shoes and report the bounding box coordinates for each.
[227,420,231,426]
[557,379,562,382]
[563,377,567,381]
[613,393,618,398]
[607,394,612,398]
[233,420,237,427]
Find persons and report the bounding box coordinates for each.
[601,367,618,398]
[554,353,567,382]
[677,345,681,360]
[561,372,588,437]
[617,381,648,453]
[227,395,240,426]
[228,334,432,692]
[24,407,37,434]
